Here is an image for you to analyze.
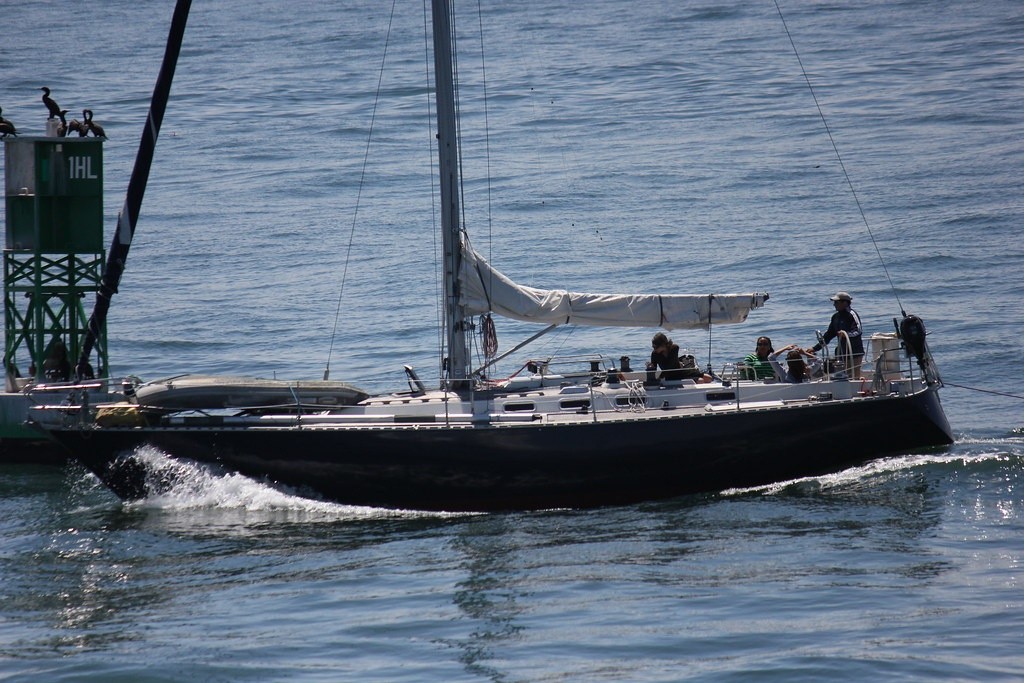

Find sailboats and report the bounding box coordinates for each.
[21,0,956,512]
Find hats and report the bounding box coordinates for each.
[830,292,852,302]
[652,332,667,349]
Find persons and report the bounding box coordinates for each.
[650,332,712,384]
[740,335,775,380]
[767,344,823,383]
[806,292,865,379]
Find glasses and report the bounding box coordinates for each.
[757,342,769,346]
